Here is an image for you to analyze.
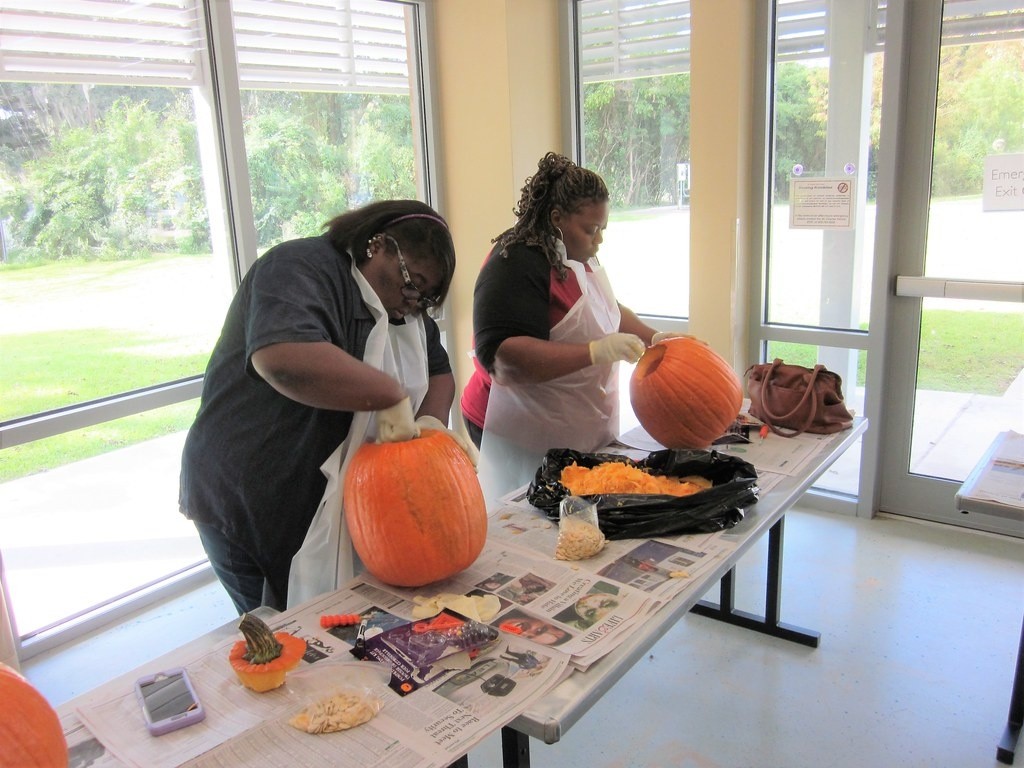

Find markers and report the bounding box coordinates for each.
[355,619,367,648]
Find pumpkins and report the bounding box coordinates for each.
[0,661,69,768]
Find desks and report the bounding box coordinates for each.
[953,431,1024,765]
[501,418,868,767]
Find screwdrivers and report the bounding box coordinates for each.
[320,613,369,627]
[758,423,768,447]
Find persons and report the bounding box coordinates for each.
[178,200,456,616]
[574,592,623,624]
[496,585,535,605]
[461,151,709,452]
[498,617,574,647]
[498,644,543,671]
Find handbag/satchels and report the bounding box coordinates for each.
[743,357,854,438]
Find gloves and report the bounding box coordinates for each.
[589,332,647,367]
[415,415,447,438]
[374,394,415,445]
[650,332,710,346]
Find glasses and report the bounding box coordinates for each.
[383,231,441,308]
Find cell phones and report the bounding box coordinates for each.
[135,666,205,737]
